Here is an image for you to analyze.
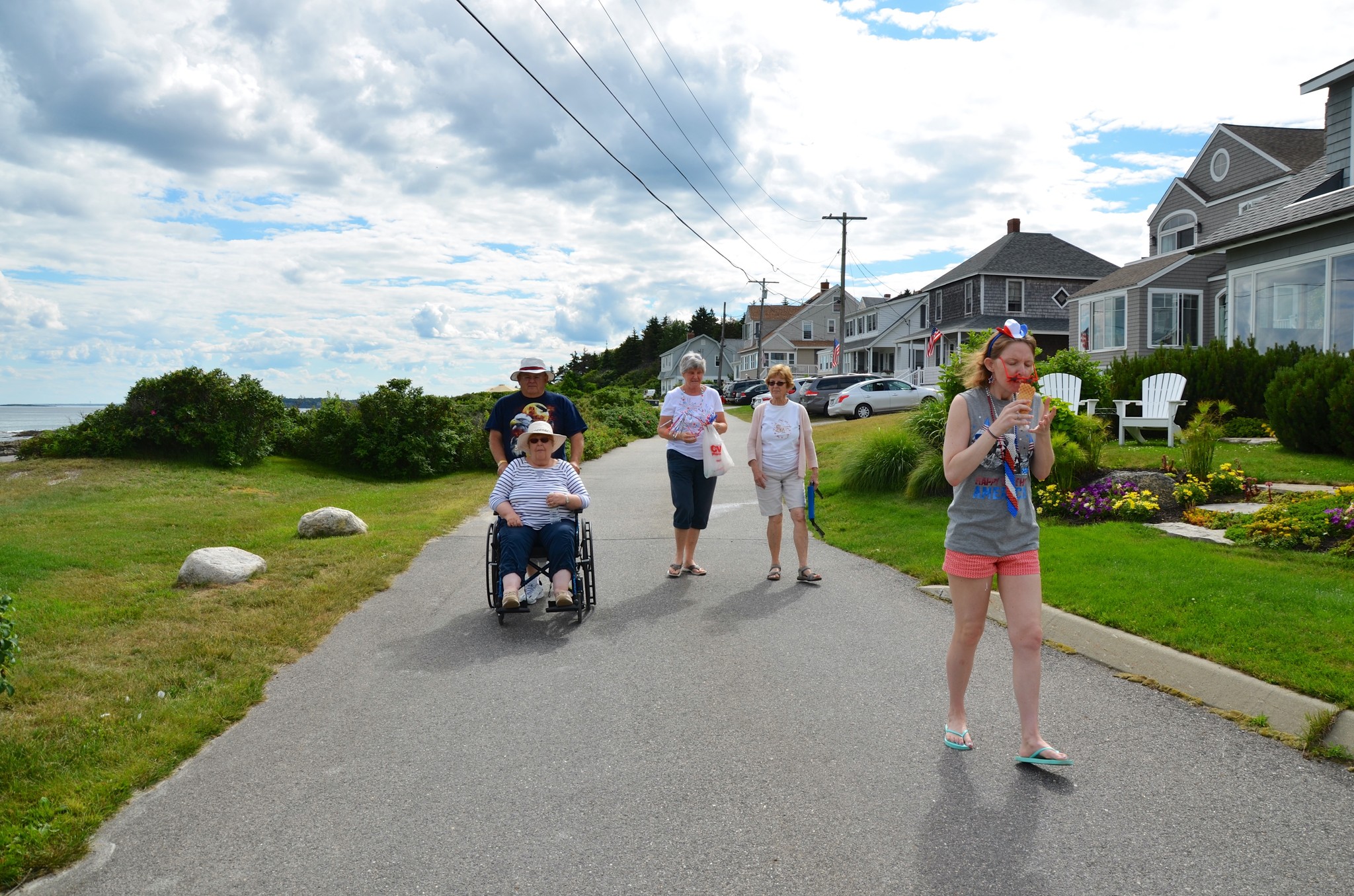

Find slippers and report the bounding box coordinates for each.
[683,565,707,575]
[1013,748,1074,766]
[667,563,682,577]
[944,724,975,751]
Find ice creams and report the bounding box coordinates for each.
[1016,383,1036,431]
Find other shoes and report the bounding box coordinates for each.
[555,592,573,607]
[501,590,521,608]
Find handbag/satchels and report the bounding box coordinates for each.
[700,424,734,479]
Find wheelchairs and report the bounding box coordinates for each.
[484,508,596,624]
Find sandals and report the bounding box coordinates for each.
[797,566,822,582]
[767,564,782,581]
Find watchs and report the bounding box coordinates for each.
[564,494,569,507]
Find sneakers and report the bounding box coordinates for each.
[520,578,545,604]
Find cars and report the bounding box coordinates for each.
[704,384,723,396]
[734,383,770,405]
[754,392,775,409]
[827,378,945,421]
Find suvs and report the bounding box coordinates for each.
[798,373,903,417]
[725,379,767,404]
[722,376,763,396]
[786,375,815,403]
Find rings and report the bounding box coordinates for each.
[552,501,554,505]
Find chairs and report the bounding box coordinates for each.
[1038,373,1100,416]
[1112,373,1188,447]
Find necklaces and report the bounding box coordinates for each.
[682,384,704,427]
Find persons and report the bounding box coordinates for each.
[942,319,1074,765]
[747,363,822,582]
[485,357,588,604]
[488,421,591,608]
[657,352,728,578]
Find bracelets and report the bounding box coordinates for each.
[987,428,998,439]
[715,423,720,430]
[673,432,677,441]
[498,460,507,467]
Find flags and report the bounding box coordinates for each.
[926,323,943,357]
[832,337,840,367]
[761,349,766,364]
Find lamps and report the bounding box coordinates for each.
[1149,235,1156,246]
[1194,221,1202,233]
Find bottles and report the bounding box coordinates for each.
[548,492,561,524]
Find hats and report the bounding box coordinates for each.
[516,421,567,454]
[996,319,1027,339]
[510,357,555,382]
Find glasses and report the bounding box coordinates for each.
[998,356,1039,385]
[528,438,553,444]
[520,374,546,380]
[766,382,788,386]
